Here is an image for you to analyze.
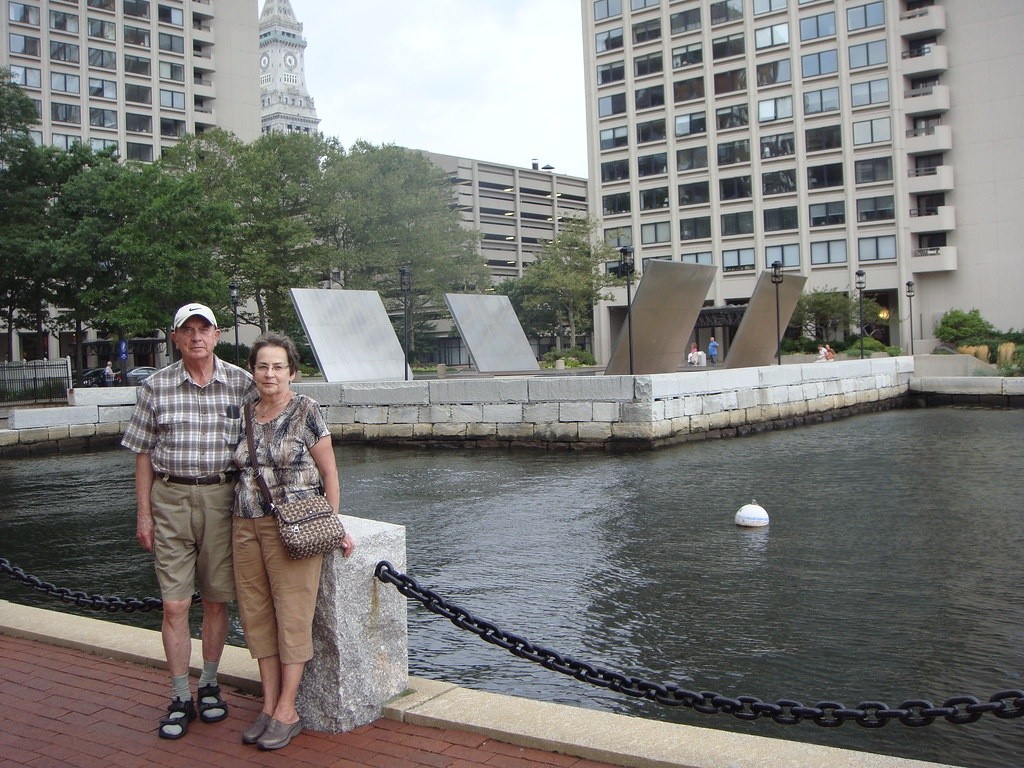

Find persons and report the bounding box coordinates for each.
[119,300,263,740]
[105,361,116,387]
[228,333,355,750]
[691,343,698,365]
[709,337,719,366]
[825,344,837,362]
[815,344,828,362]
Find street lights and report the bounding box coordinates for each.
[770,259,782,366]
[905,281,915,355]
[618,245,635,374]
[227,281,241,367]
[398,265,413,379]
[855,270,867,359]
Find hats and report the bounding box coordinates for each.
[174,302,217,333]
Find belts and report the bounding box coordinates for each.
[156,469,239,486]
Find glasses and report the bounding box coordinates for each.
[254,363,292,373]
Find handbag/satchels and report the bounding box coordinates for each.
[272,495,345,559]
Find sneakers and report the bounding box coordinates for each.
[256,717,303,751]
[242,711,272,743]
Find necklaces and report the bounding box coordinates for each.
[260,393,286,417]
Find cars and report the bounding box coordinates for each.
[71,365,158,387]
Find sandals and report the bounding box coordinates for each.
[197,684,227,722]
[159,695,196,739]
